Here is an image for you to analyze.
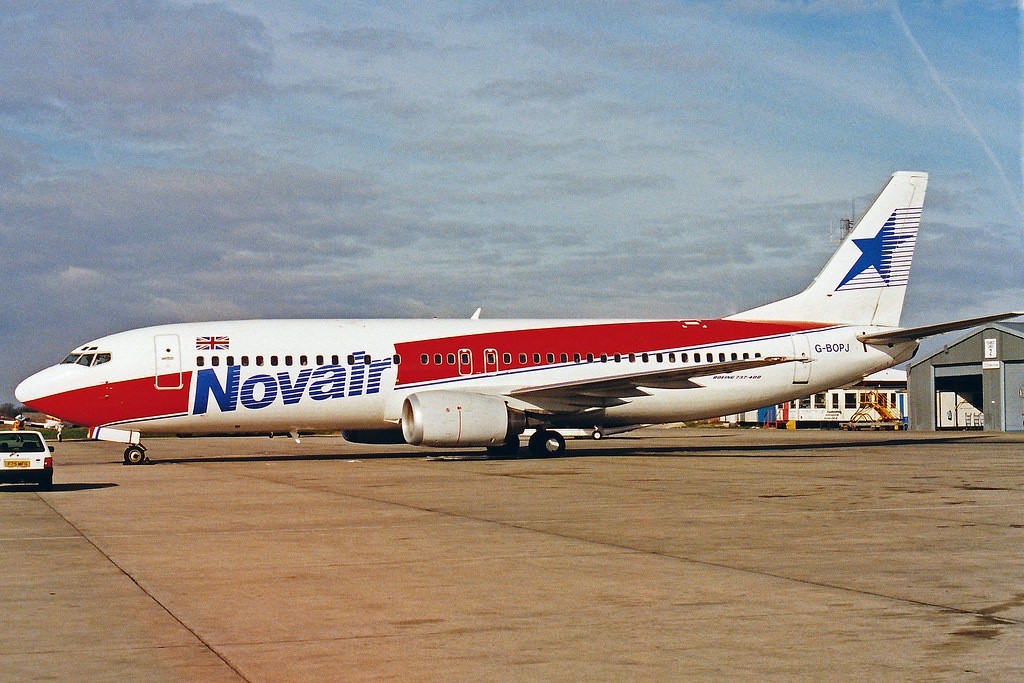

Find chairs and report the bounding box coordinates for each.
[23,442,37,448]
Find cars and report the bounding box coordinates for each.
[0,431,55,488]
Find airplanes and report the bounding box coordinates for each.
[14,173,1024,466]
